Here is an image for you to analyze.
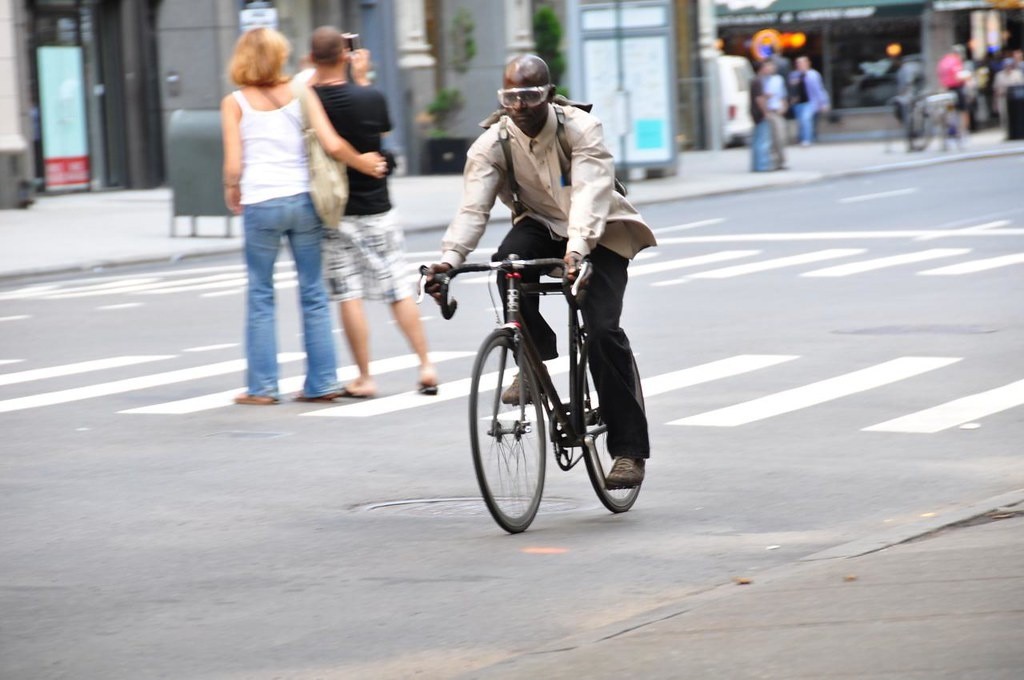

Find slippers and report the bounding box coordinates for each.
[292,390,335,405]
[339,388,371,398]
[418,378,438,394]
[235,393,278,406]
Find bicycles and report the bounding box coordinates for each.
[416,253,643,534]
[904,94,970,152]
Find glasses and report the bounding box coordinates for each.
[496,86,551,108]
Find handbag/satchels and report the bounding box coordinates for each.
[304,128,349,230]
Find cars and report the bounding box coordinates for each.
[716,55,758,148]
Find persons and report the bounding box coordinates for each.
[748,55,832,173]
[936,25,1024,139]
[416,54,657,490]
[219,25,438,405]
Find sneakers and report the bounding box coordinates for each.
[605,455,645,486]
[502,364,550,405]
